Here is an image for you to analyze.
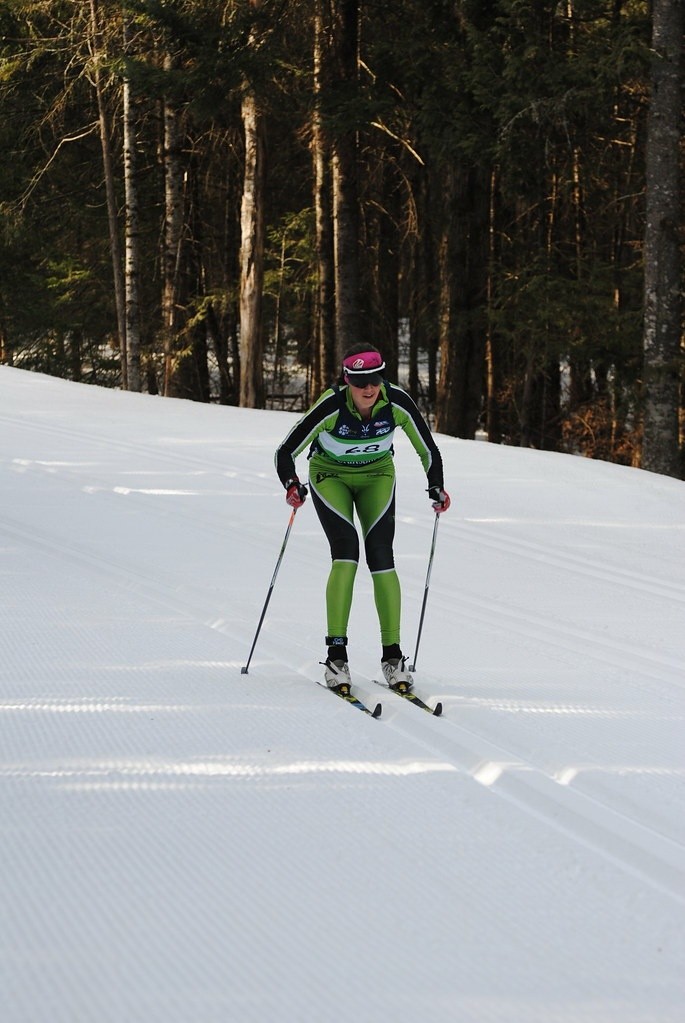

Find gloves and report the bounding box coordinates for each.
[432,487,450,513]
[284,477,308,507]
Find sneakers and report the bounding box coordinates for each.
[319,659,351,690]
[381,657,413,689]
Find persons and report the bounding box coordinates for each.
[274,341,451,695]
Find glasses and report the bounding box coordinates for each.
[343,362,386,388]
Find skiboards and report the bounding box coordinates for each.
[314,679,442,719]
